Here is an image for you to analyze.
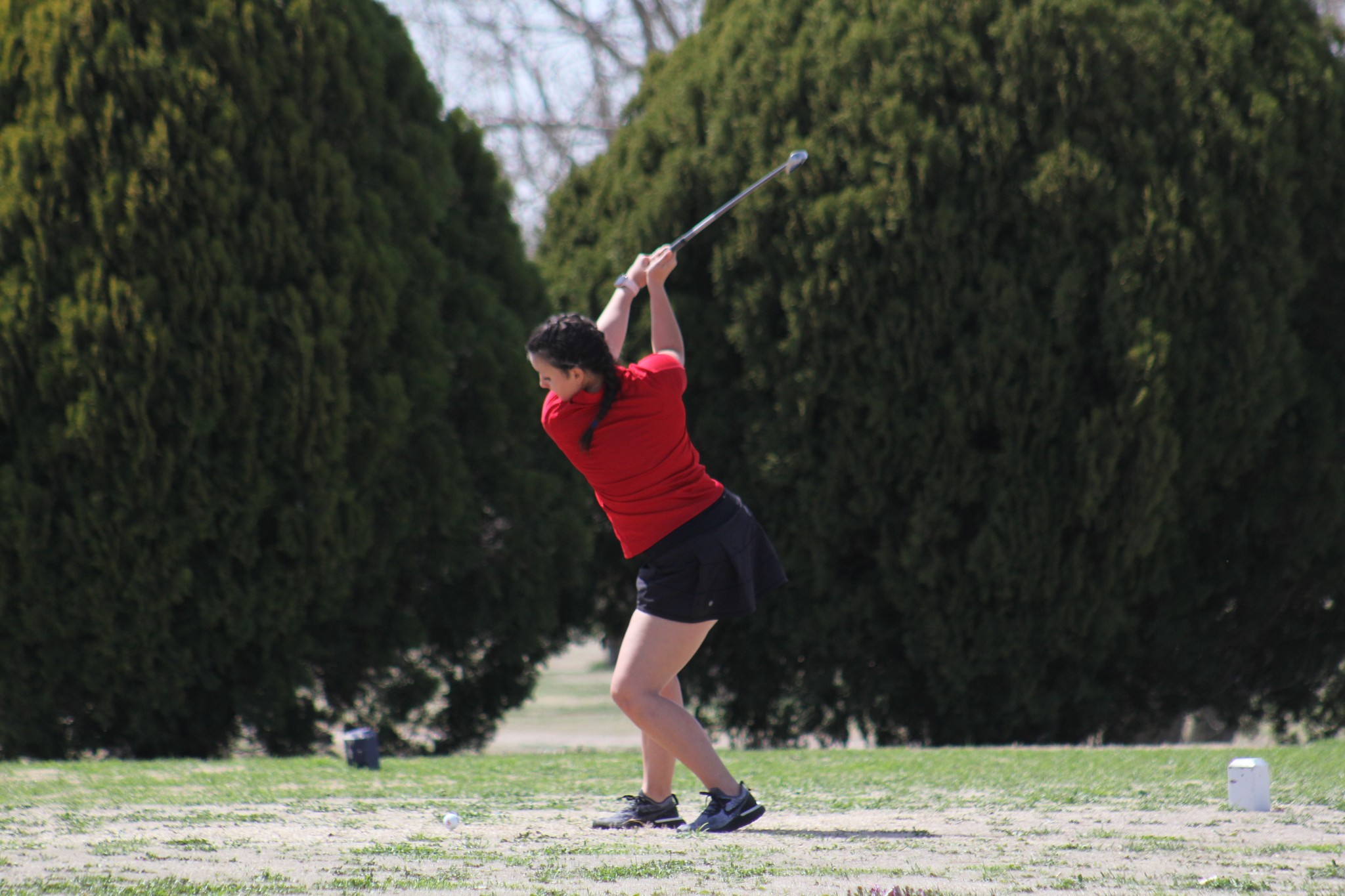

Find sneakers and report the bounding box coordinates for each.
[676,781,765,833]
[593,789,686,830]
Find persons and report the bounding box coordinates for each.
[525,243,788,832]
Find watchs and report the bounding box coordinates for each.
[614,275,640,297]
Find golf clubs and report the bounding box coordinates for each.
[666,147,810,254]
